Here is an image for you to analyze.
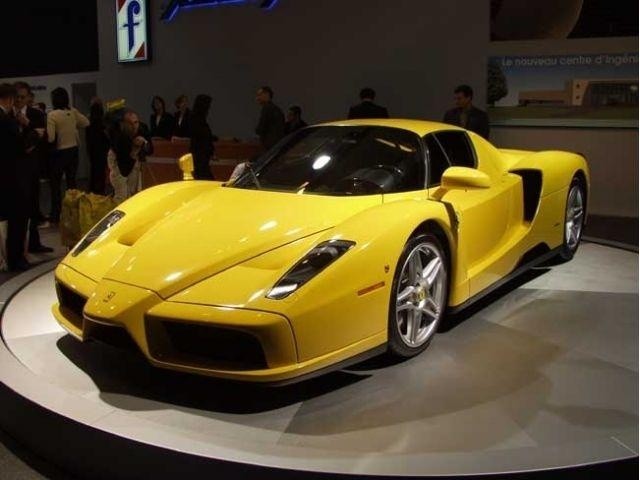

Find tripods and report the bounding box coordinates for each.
[136,153,157,193]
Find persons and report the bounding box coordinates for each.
[254,86,285,145]
[348,88,389,119]
[284,106,308,134]
[443,84,490,140]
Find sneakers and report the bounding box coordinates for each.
[8,259,39,272]
[26,243,55,255]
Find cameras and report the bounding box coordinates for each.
[141,140,151,153]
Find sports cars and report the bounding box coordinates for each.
[48,111,590,396]
